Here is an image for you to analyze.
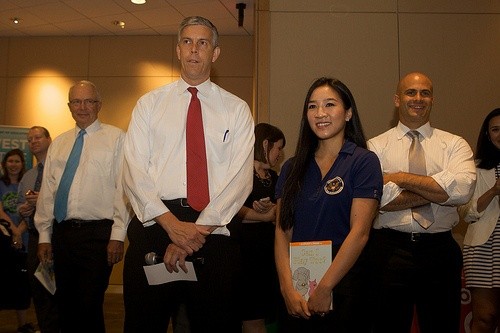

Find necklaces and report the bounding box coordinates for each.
[496,164,499,177]
[255,167,270,185]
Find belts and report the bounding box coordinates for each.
[162,198,190,207]
[372,228,451,241]
[53,219,113,227]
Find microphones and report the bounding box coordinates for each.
[145,251,204,266]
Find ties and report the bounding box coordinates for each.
[29,163,44,227]
[186,87,210,211]
[407,130,434,229]
[54,130,87,223]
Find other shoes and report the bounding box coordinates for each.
[17,322,40,333]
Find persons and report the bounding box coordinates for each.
[235,77,384,333]
[0,126,55,333]
[366,72,477,333]
[462,108,500,333]
[34,80,135,333]
[122,16,255,333]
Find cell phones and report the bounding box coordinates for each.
[25,190,36,196]
[259,196,271,205]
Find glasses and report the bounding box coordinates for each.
[70,100,98,105]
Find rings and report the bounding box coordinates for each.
[320,313,324,317]
[14,242,16,244]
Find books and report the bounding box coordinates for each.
[289,241,333,310]
[35,263,57,295]
